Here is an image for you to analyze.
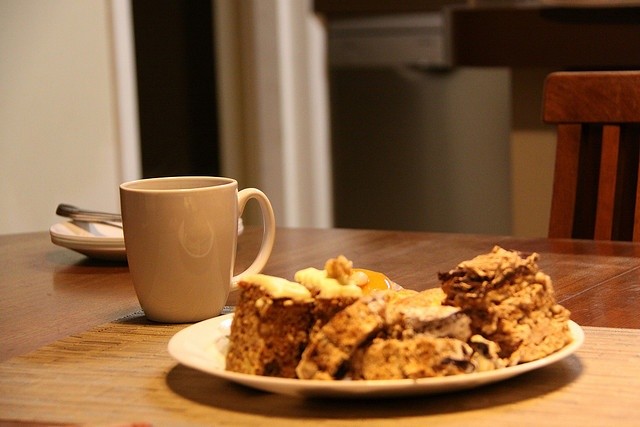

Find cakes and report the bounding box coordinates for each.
[225,273,315,378]
[361,332,492,380]
[294,298,385,380]
[437,245,574,366]
[383,288,470,341]
[293,254,399,299]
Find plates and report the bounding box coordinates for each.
[50,218,246,263]
[166,312,585,400]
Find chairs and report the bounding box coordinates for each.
[542,71,638,241]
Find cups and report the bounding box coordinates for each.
[119,174,277,324]
